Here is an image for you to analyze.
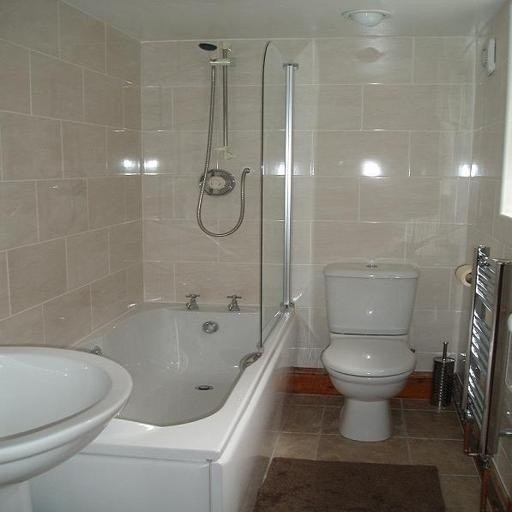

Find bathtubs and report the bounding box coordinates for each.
[30,304,299,512]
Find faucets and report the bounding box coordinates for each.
[227,295,242,313]
[186,295,200,310]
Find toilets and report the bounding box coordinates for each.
[322,263,420,442]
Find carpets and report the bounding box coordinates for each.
[254,457,447,512]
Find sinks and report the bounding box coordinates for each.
[2,343,132,487]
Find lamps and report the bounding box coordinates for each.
[341,8,391,27]
